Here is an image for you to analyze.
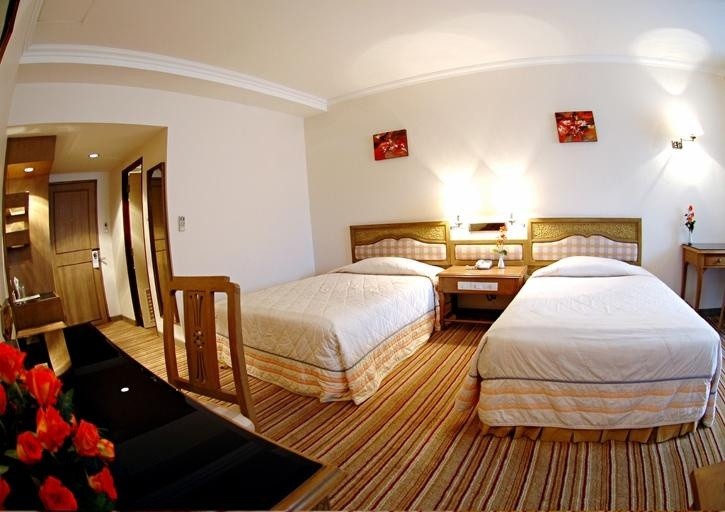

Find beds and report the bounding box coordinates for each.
[214,221,451,405]
[454,217,721,442]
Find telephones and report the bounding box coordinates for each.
[475,259,493,270]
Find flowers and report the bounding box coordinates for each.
[0,338,117,511]
[493,225,507,256]
[685,206,696,233]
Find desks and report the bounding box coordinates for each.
[0,319,351,511]
[681,243,725,314]
[437,264,527,329]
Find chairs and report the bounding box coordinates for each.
[163,275,258,432]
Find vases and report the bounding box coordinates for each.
[689,232,693,244]
[498,255,504,269]
